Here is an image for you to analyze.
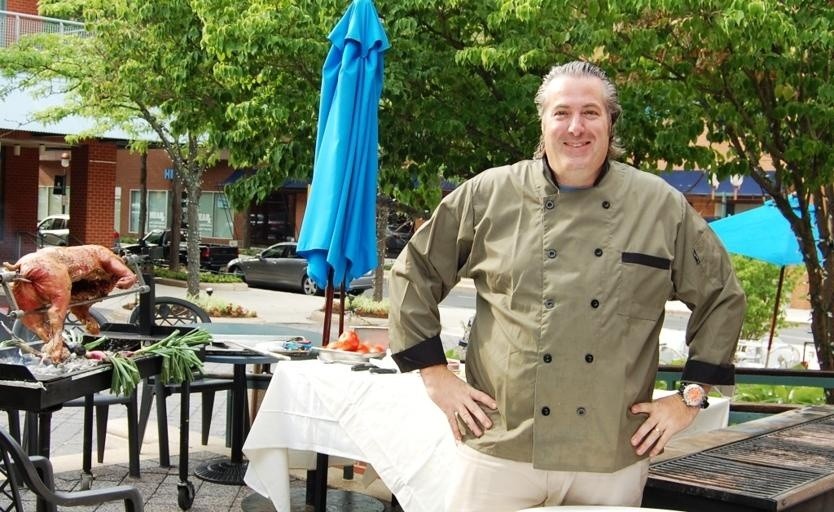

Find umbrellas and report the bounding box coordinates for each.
[707,194,830,367]
[297,0,384,346]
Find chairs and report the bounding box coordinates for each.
[0,296,238,511]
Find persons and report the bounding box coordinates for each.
[387,60,745,512]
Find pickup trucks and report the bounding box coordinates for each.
[119,228,236,273]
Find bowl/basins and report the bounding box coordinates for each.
[310,346,387,365]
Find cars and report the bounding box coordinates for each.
[227,241,373,295]
[36,214,72,246]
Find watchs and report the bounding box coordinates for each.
[678,384,709,409]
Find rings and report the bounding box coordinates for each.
[655,426,662,435]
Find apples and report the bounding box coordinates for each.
[326,331,385,353]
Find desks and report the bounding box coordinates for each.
[204,349,733,512]
[240,349,733,512]
